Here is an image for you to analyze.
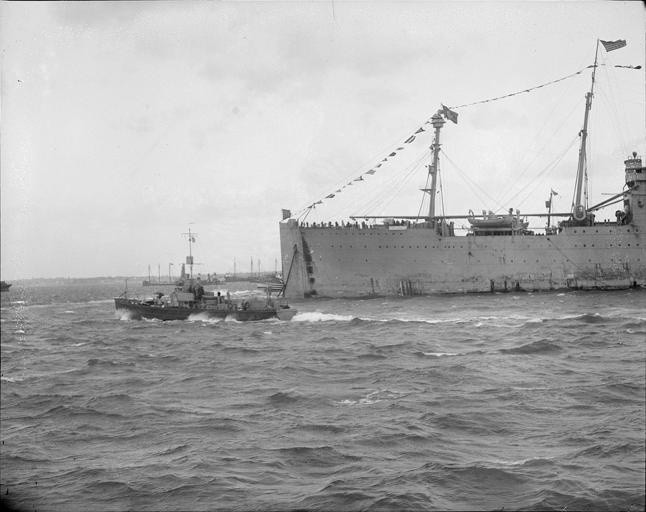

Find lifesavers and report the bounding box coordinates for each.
[574,204,586,221]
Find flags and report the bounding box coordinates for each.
[444,106,459,124]
[601,39,627,51]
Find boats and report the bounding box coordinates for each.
[0,280,11,292]
[113,228,298,323]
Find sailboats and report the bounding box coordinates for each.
[141,257,279,287]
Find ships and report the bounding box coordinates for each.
[278,39,646,301]
